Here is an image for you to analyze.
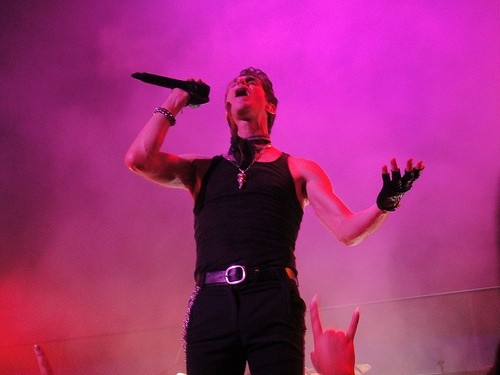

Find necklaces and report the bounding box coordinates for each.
[224,143,275,189]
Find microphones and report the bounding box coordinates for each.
[131,72,210,98]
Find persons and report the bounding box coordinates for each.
[125,66,426,375]
[32,343,54,375]
[309,297,360,375]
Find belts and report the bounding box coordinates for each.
[194,266,298,288]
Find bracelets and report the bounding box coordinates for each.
[153,106,177,126]
